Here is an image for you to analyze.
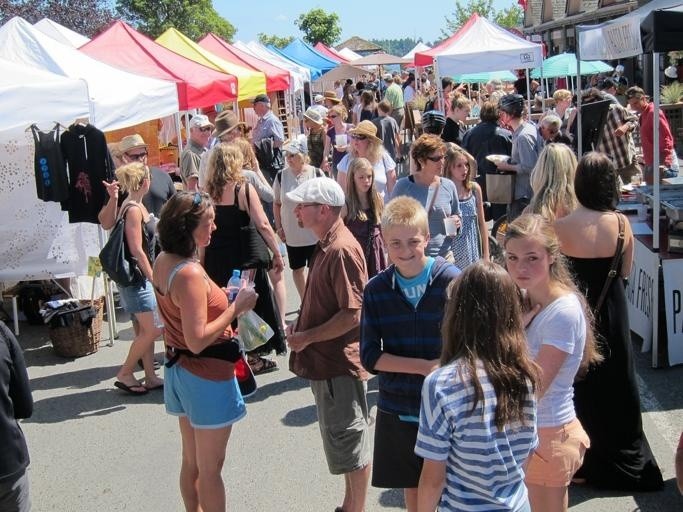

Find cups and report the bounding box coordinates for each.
[442,217,457,237]
[630,173,643,188]
[335,135,346,146]
[636,205,648,221]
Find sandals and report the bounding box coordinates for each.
[248,358,276,372]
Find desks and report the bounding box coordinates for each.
[608,194,682,374]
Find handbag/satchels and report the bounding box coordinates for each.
[240,225,270,269]
[99,204,149,284]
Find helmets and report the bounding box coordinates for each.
[499,94,523,114]
[421,110,445,127]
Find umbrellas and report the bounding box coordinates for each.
[348,48,411,101]
[528,50,614,94]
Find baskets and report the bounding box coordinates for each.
[47,299,104,357]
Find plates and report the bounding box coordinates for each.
[485,154,510,164]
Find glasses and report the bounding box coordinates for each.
[200,128,210,131]
[125,152,146,159]
[352,134,366,140]
[427,157,441,161]
[192,192,201,208]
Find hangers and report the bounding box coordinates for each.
[24,118,90,133]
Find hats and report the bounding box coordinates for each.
[349,120,381,144]
[251,94,269,103]
[315,92,340,103]
[303,110,323,125]
[626,86,650,98]
[189,110,246,137]
[286,177,345,206]
[281,140,307,155]
[116,134,148,156]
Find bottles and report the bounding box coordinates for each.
[224,269,241,310]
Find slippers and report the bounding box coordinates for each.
[114,382,147,394]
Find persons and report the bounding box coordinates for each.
[0,321,33,511]
[286,175,373,509]
[358,193,464,510]
[500,210,593,510]
[414,260,541,512]
[148,186,258,511]
[94,68,681,493]
